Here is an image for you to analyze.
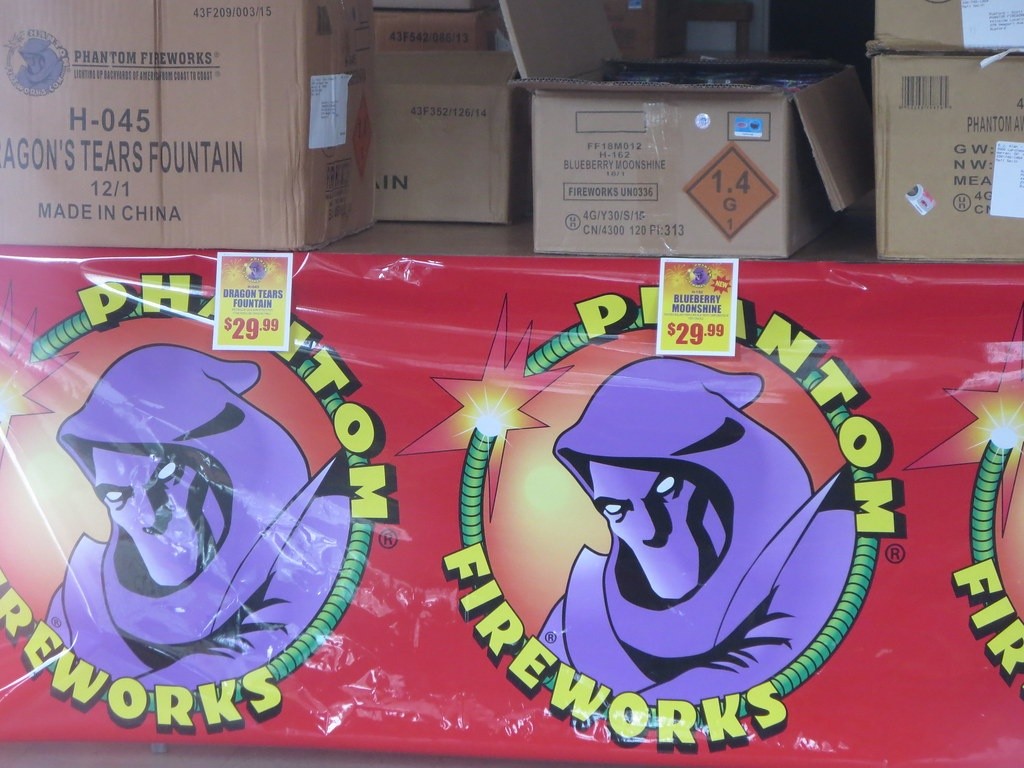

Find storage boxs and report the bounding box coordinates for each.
[0,0,1024,263]
